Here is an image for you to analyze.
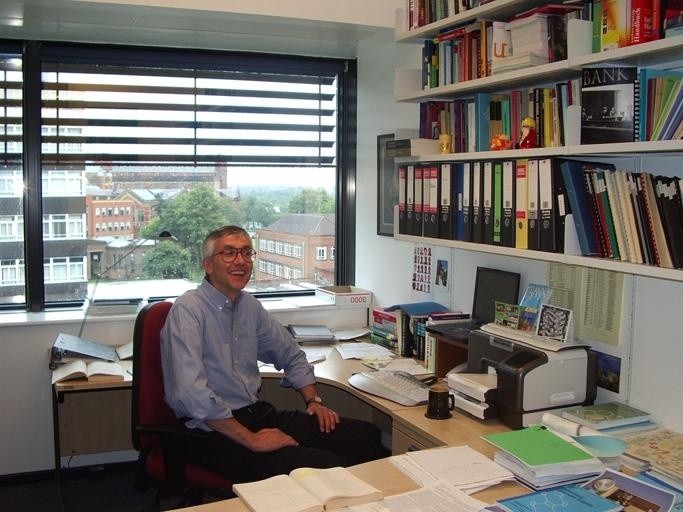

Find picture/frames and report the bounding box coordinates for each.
[377,132,410,237]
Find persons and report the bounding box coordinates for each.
[159,222,387,484]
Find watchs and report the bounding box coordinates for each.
[302,395,323,405]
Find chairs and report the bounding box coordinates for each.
[131,301,236,512]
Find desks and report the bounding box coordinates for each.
[52,344,538,512]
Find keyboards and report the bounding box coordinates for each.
[348,369,430,407]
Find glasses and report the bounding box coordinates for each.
[212,247,256,263]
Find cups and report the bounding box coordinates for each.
[439,134,452,154]
[426,385,456,419]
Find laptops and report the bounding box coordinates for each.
[426,266,520,344]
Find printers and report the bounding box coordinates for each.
[447,329,598,429]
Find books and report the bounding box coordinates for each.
[283,300,471,374]
[419,66,682,153]
[407,0,683,91]
[398,156,683,272]
[48,332,133,382]
[371,400,682,511]
[231,464,384,511]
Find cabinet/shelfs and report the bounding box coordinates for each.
[395,0,683,283]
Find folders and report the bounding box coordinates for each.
[398,159,681,269]
[50,333,116,363]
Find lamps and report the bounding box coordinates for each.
[78,230,172,339]
